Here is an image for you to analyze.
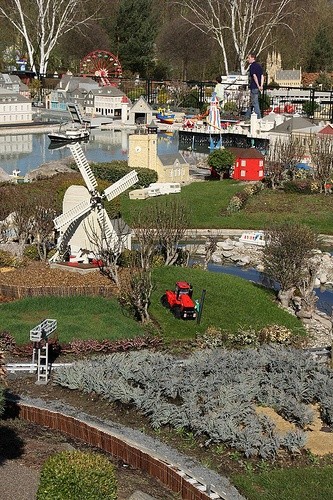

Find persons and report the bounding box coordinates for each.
[245,53,264,124]
[214,76,225,107]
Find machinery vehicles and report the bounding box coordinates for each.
[164,281,198,320]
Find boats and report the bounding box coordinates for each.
[157,109,209,126]
[47,115,90,144]
